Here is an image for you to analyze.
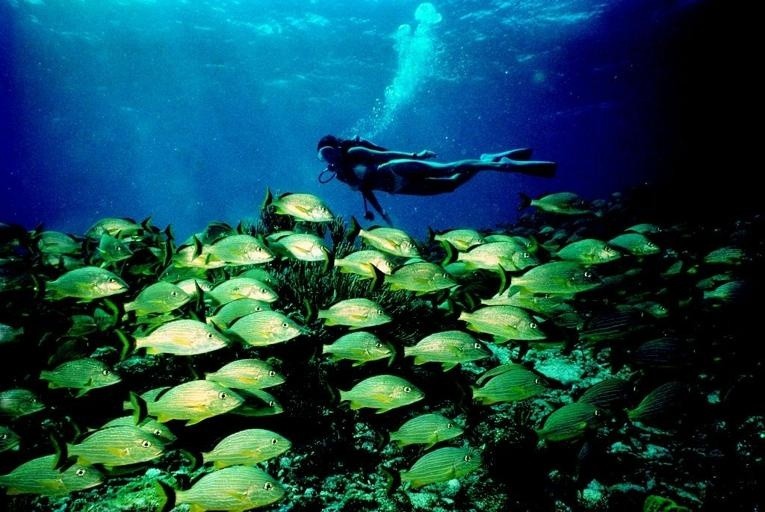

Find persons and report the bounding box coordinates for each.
[315,134,556,229]
